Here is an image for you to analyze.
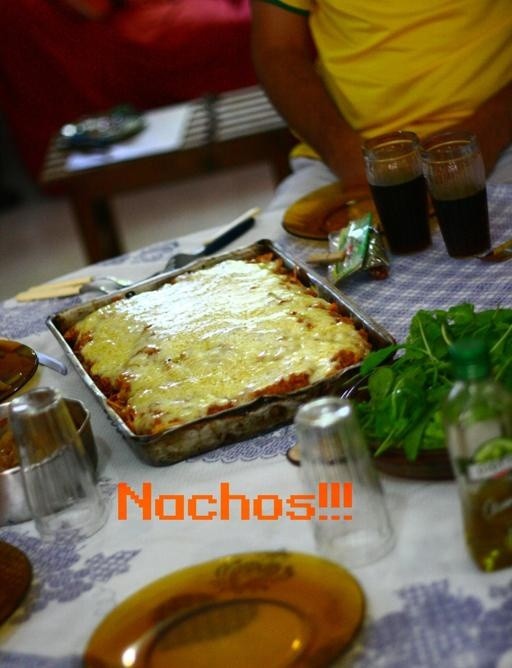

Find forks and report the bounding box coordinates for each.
[16,215,257,300]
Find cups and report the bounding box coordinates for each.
[9,386,110,540]
[359,129,492,259]
[293,394,399,569]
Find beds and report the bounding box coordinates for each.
[0,180,512,667]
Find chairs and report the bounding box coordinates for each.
[0,0,259,197]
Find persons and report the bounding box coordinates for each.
[249,0,512,220]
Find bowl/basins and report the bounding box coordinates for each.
[0,396,97,526]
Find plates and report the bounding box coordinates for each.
[0,339,38,403]
[2,540,32,626]
[64,114,142,148]
[84,552,366,666]
[282,179,435,239]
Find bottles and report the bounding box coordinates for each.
[444,338,511,572]
